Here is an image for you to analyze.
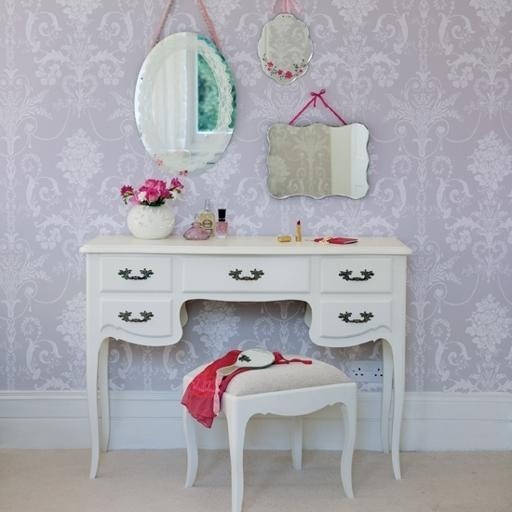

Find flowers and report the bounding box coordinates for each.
[120,177,184,206]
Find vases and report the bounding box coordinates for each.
[126,204,176,239]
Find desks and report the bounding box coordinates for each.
[79,231,414,482]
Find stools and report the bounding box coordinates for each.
[181,353,357,512]
